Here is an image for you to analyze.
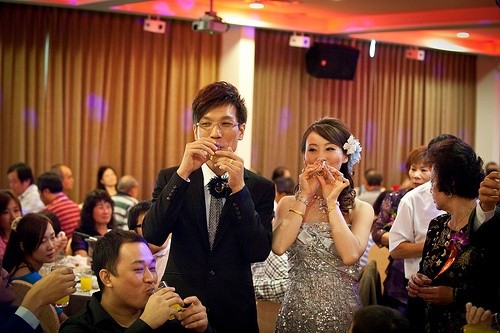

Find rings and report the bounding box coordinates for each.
[406,286,410,291]
[230,159,233,164]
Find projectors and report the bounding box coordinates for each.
[192,20,229,33]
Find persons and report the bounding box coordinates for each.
[3,213,69,326]
[0,163,172,267]
[57,228,208,333]
[355,134,500,333]
[142,81,275,333]
[0,264,76,333]
[272,118,375,333]
[251,168,294,305]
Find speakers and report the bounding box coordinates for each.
[306,43,360,81]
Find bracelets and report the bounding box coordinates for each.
[295,191,310,208]
[289,208,303,217]
[327,202,340,214]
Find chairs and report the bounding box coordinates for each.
[12,280,61,333]
[346,243,390,333]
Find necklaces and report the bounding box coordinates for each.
[447,210,471,239]
[312,193,329,213]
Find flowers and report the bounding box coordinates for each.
[382,207,397,229]
[343,135,362,175]
[441,230,469,258]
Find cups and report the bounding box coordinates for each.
[51,266,69,307]
[209,143,220,160]
[461,313,499,333]
[151,281,182,320]
[317,160,329,176]
[80,266,92,292]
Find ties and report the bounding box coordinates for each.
[207,176,232,250]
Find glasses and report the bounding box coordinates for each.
[196,120,240,131]
